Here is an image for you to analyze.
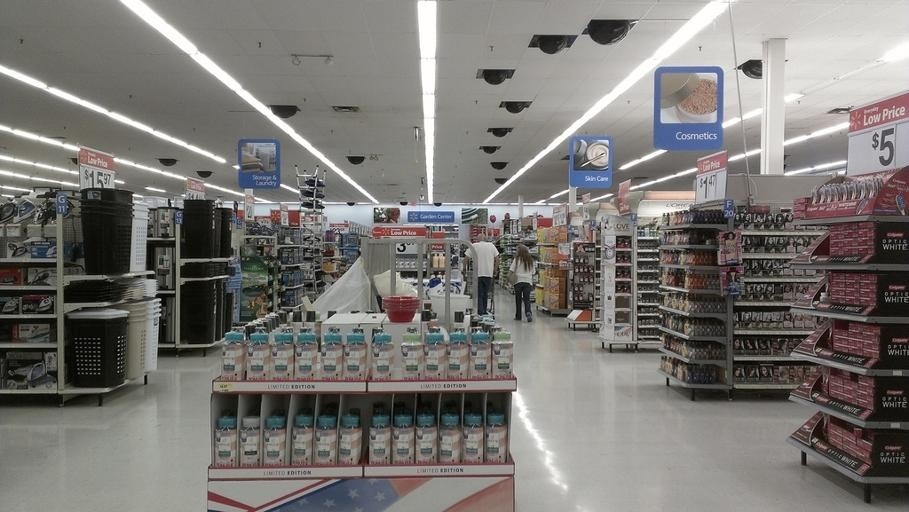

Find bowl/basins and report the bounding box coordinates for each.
[382,296,420,323]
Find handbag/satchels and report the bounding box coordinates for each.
[508,272,518,284]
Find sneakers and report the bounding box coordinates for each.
[526,311,532,322]
[514,317,522,321]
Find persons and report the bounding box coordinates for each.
[462,234,501,315]
[506,245,535,322]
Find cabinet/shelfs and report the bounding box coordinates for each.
[148,197,245,359]
[3,187,161,411]
[202,233,518,511]
[493,211,664,352]
[784,168,909,503]
[656,201,824,405]
[234,220,368,332]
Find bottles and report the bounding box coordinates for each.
[423,333,447,381]
[319,333,344,380]
[468,333,492,382]
[293,333,319,383]
[270,332,295,383]
[263,415,286,468]
[463,414,485,464]
[439,414,462,465]
[342,334,368,382]
[416,415,438,467]
[368,414,391,466]
[313,415,337,466]
[220,331,246,382]
[238,416,260,467]
[338,415,363,466]
[399,333,425,382]
[289,414,314,467]
[370,334,395,383]
[485,414,509,464]
[447,331,470,381]
[214,417,239,468]
[392,415,415,465]
[244,333,269,382]
[490,331,515,380]
